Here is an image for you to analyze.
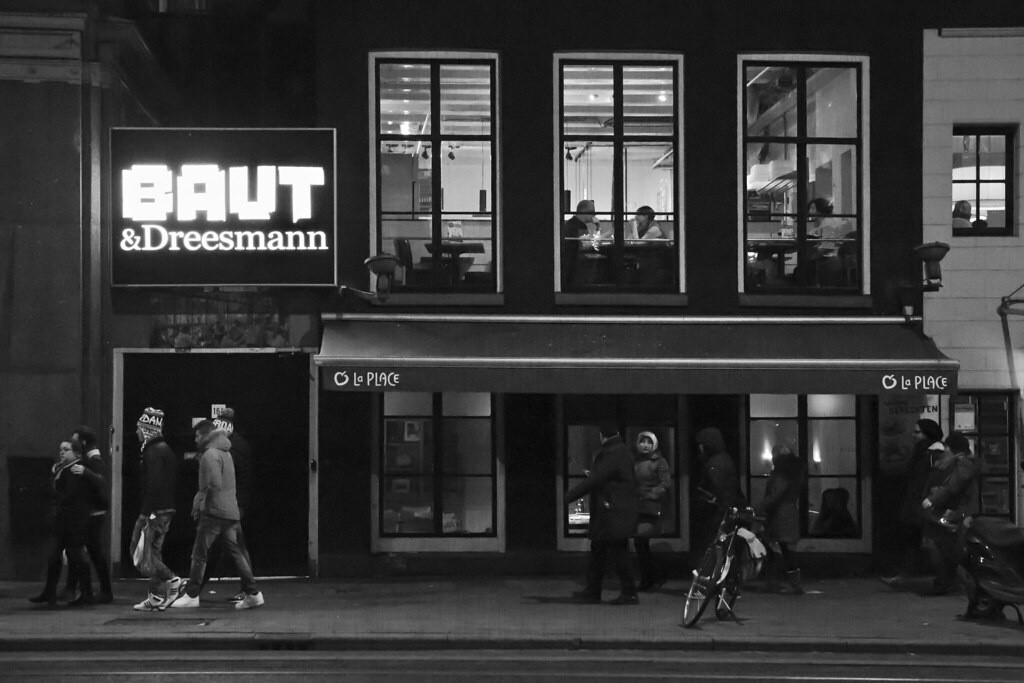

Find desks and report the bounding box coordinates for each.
[425,242,485,290]
[747,236,856,293]
[598,242,652,285]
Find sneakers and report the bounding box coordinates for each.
[133,592,167,611]
[235,591,264,609]
[171,594,200,608]
[161,577,188,607]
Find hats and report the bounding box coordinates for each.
[212,408,234,436]
[137,407,164,453]
[941,431,969,453]
[918,418,943,441]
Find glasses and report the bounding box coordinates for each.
[914,429,920,434]
[58,447,75,453]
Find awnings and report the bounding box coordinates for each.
[313,313,960,398]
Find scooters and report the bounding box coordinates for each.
[957,512,1024,625]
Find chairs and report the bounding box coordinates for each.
[463,260,496,292]
[394,237,422,286]
[819,230,859,283]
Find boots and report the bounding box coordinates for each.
[786,569,804,595]
[767,565,786,594]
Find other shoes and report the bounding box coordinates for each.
[956,610,982,622]
[574,590,601,602]
[29,590,58,604]
[68,591,95,608]
[90,591,113,604]
[880,574,912,592]
[604,593,638,604]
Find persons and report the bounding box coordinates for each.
[564,418,639,607]
[810,488,855,535]
[681,427,740,600]
[561,200,614,285]
[628,431,674,591]
[29,437,96,607]
[951,201,972,228]
[130,406,187,613]
[54,426,113,606]
[169,408,264,609]
[622,207,669,287]
[750,445,807,595]
[787,198,848,283]
[882,416,981,596]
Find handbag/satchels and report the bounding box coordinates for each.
[130,514,155,577]
[735,528,768,581]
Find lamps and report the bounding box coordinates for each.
[565,147,577,160]
[448,145,460,160]
[899,241,950,291]
[422,144,431,159]
[362,255,400,304]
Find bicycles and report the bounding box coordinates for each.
[682,487,756,628]
